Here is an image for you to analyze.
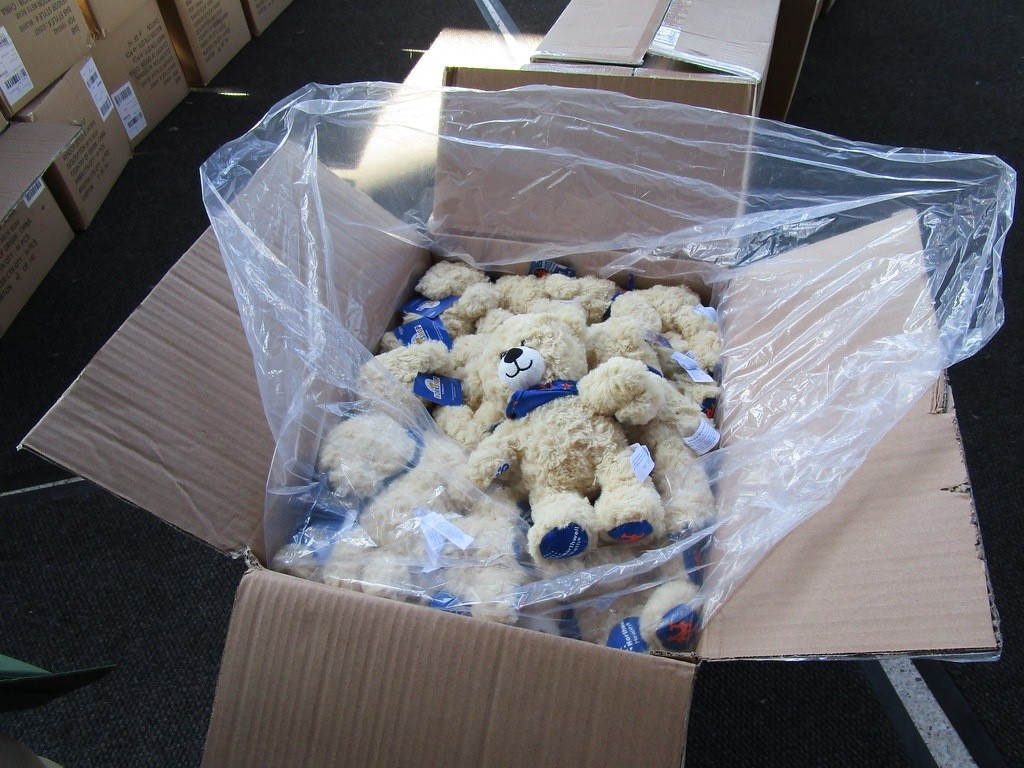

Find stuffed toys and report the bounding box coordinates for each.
[272,260,723,654]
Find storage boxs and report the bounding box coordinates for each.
[1,1,1004,768]
